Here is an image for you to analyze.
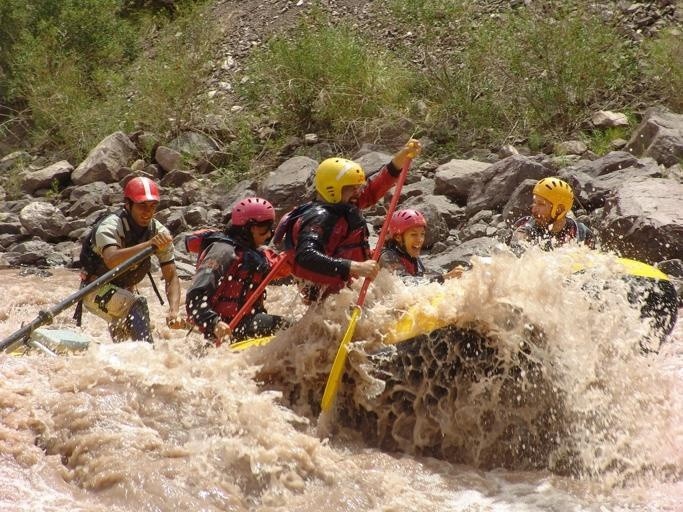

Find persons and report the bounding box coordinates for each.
[508,175,595,257]
[377,207,464,289]
[78,176,181,342]
[184,197,291,342]
[270,138,422,307]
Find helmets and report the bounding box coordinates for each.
[231,197,276,226]
[124,176,161,204]
[533,177,574,213]
[314,157,366,205]
[389,209,428,240]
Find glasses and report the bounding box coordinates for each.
[249,221,273,231]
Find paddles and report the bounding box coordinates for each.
[321,142,417,411]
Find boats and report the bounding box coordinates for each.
[23,252,679,422]
